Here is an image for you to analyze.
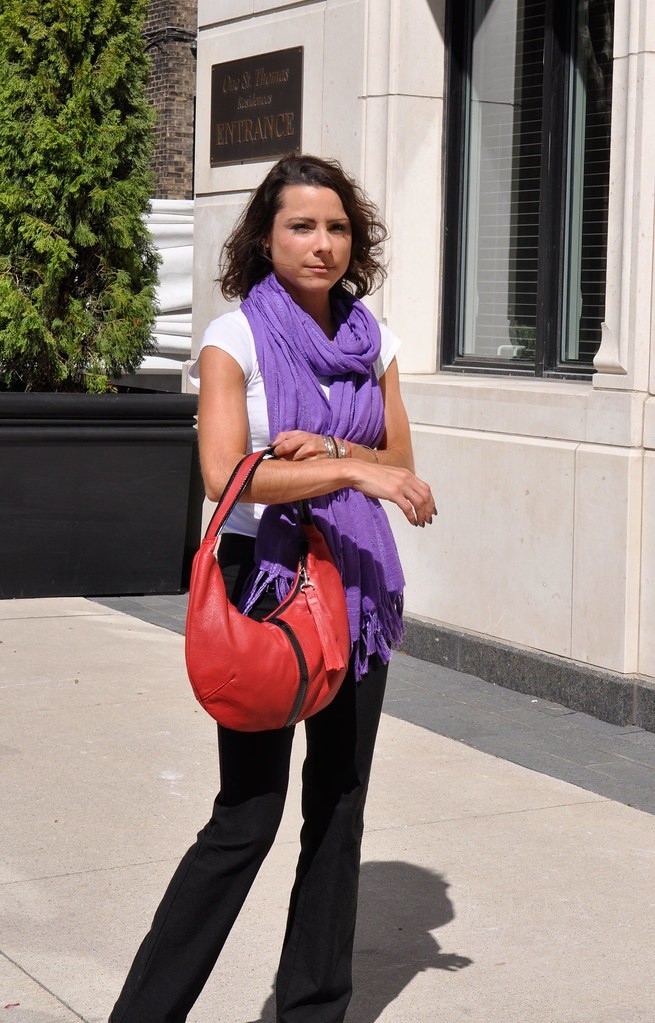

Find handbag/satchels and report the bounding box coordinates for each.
[185,443,353,731]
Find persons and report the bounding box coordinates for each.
[109,150,438,1023]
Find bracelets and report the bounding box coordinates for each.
[344,440,353,458]
[321,432,336,459]
[361,443,379,463]
[338,437,344,459]
[329,434,339,460]
